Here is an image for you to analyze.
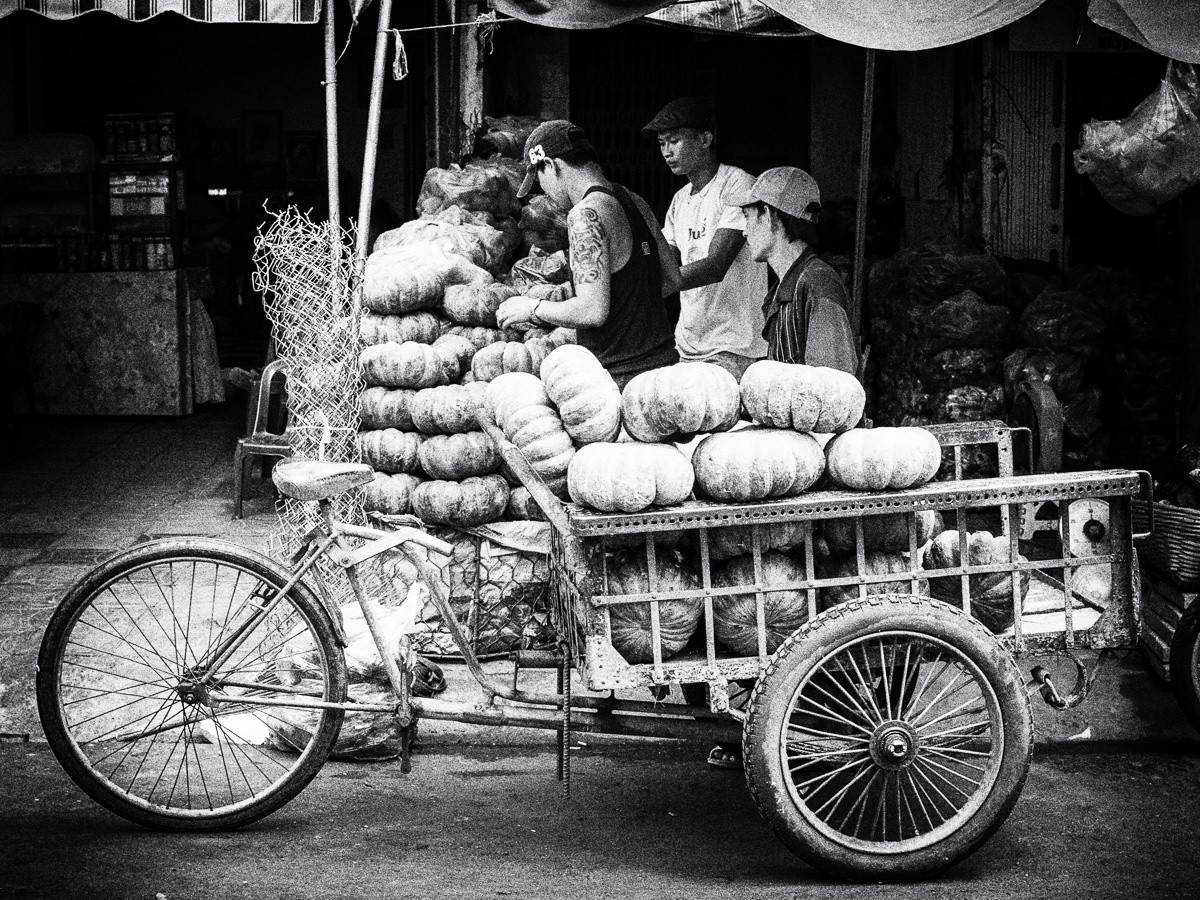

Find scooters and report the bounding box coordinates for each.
[1133,443,1200,731]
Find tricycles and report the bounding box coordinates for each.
[35,408,1158,888]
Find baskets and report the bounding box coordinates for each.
[1132,499,1200,582]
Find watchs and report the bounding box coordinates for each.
[527,298,545,325]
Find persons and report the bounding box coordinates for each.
[640,101,772,385]
[707,167,860,767]
[495,118,682,394]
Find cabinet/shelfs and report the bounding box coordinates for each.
[90,110,183,269]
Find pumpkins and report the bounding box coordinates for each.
[352,221,577,530]
[481,343,1033,662]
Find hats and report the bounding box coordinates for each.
[722,166,822,224]
[516,120,589,198]
[640,97,716,133]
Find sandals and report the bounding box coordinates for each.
[709,745,738,768]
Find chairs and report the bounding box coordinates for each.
[235,355,306,523]
